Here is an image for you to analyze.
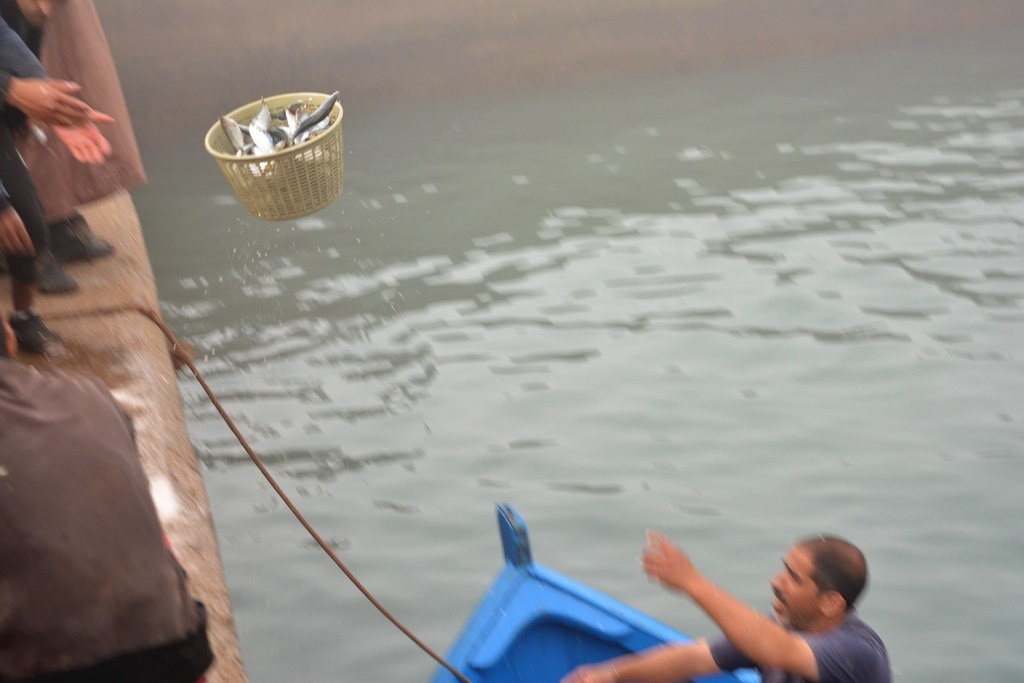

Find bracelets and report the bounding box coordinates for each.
[610,662,622,682]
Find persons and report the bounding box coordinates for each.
[560,530,893,683]
[0,0,218,682]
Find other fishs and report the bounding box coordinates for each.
[219,115,245,152]
[234,91,340,178]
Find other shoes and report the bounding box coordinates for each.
[30,251,78,293]
[9,311,63,354]
[48,216,112,261]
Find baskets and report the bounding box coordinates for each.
[204,91,343,221]
